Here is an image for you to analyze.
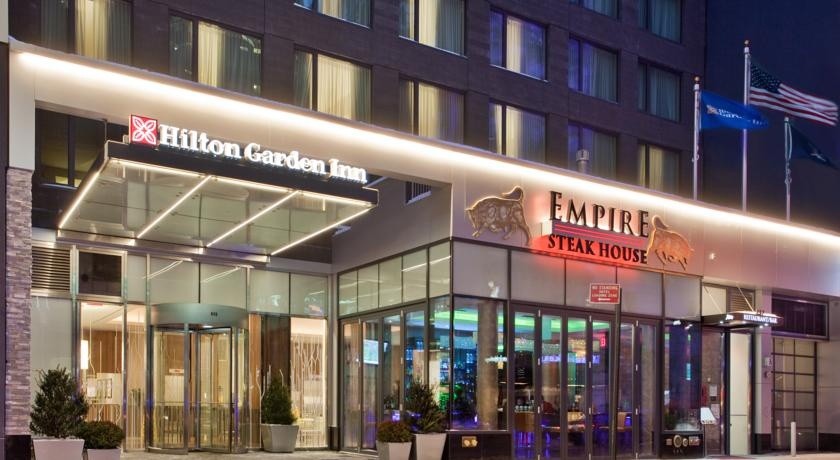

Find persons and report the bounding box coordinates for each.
[452,389,474,422]
[516,396,555,412]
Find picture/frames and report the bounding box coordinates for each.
[96,378,112,399]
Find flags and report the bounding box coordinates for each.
[746,61,838,126]
[697,90,769,129]
[787,124,837,169]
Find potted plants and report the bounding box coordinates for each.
[375,420,413,460]
[398,373,448,460]
[27,363,85,460]
[260,378,300,454]
[81,420,125,460]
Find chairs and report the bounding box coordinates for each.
[516,411,632,449]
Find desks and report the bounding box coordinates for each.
[599,425,632,433]
[545,424,596,447]
